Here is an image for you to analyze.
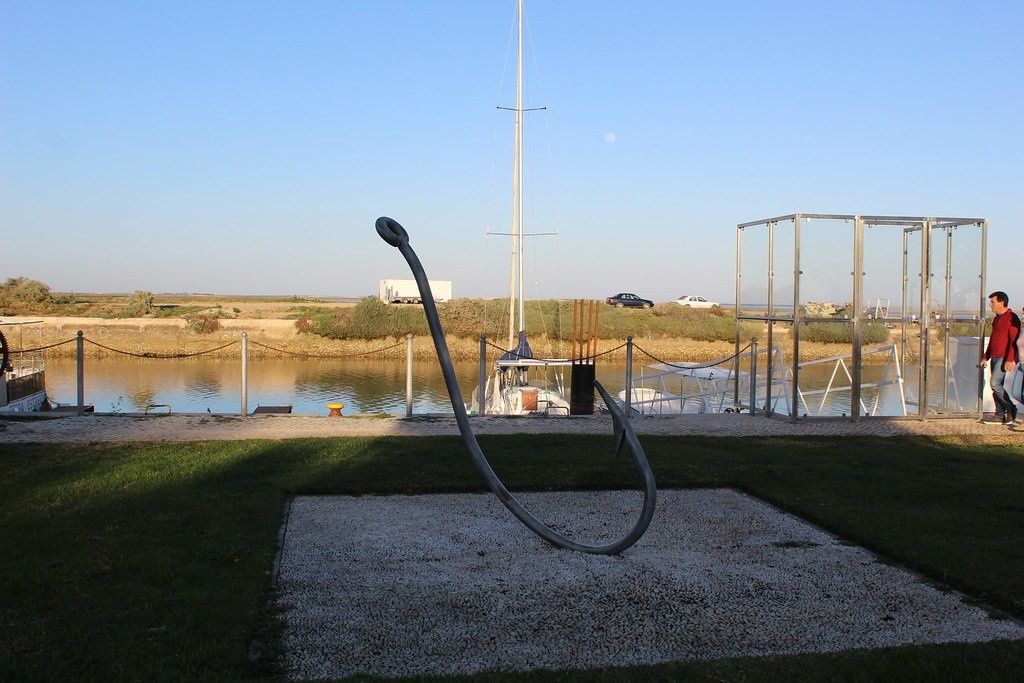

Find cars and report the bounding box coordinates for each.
[605,293,654,309]
[671,295,720,309]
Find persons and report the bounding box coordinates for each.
[979,291,1024,432]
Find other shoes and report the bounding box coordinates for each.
[1013,423,1024,431]
[982,416,1004,424]
[1002,405,1018,425]
